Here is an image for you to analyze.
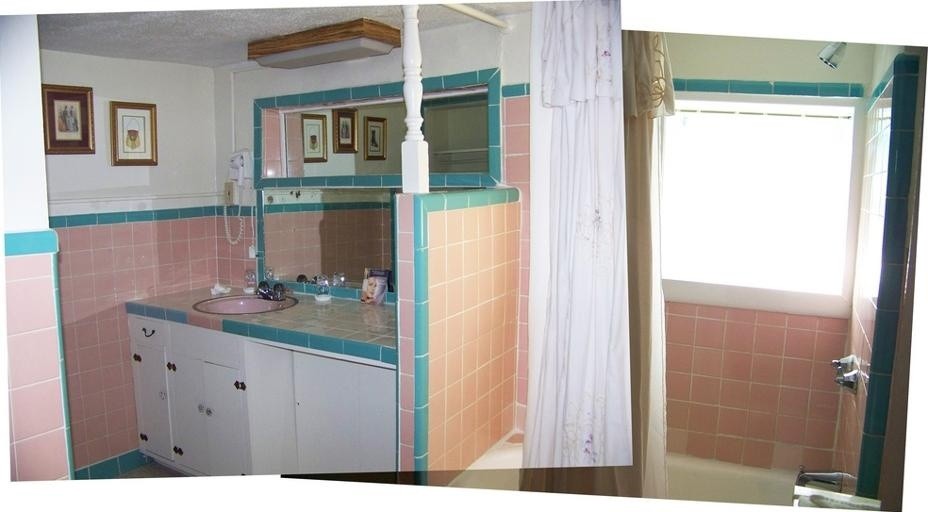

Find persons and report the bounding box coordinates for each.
[361,276,378,304]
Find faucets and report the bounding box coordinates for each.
[255,288,273,299]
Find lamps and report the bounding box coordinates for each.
[248,18,400,70]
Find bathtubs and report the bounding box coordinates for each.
[449,429,839,506]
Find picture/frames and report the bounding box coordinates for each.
[364,115,388,160]
[332,109,359,153]
[110,101,158,166]
[42,83,95,155]
[301,114,327,163]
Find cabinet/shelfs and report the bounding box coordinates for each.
[248,323,398,474]
[125,302,248,476]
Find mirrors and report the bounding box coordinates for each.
[256,188,397,306]
[253,68,502,188]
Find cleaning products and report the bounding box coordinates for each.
[313,275,332,304]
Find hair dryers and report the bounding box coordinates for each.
[225,155,244,187]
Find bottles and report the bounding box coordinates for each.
[315,279,331,301]
